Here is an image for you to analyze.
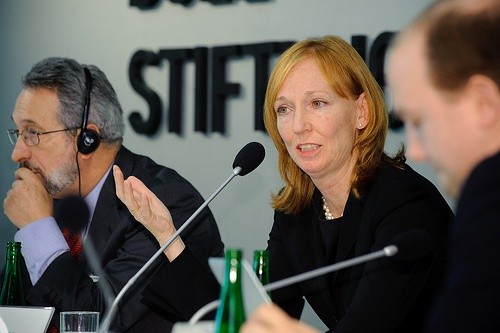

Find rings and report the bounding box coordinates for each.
[130,205,139,212]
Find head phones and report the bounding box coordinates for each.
[76,66,101,156]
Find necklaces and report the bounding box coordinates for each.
[321,196,335,220]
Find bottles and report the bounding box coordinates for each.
[215,249,247,333]
[252,250,271,300]
[0,242,24,307]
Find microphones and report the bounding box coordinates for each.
[97,142,265,333]
[189,228,433,326]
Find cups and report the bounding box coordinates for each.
[60,311,99,333]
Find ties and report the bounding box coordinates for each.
[61,227,83,256]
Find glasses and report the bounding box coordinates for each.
[7,127,107,146]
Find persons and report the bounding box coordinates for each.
[241,0,500,333]
[0,57,226,333]
[112,36,455,330]
